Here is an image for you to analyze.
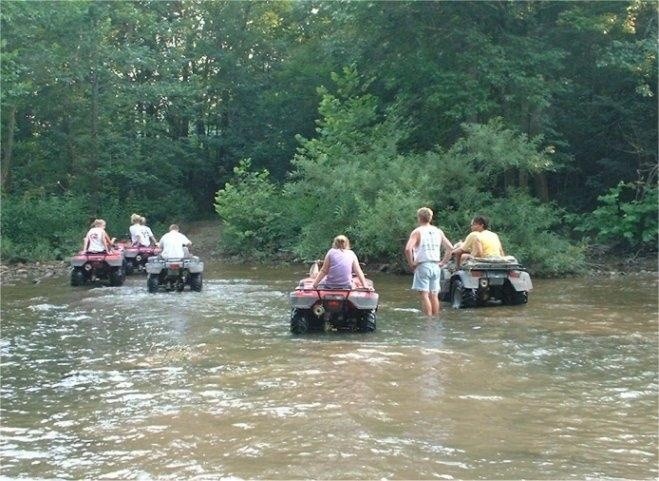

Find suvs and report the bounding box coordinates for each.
[438,242,533,310]
[289,259,379,335]
[124,241,160,275]
[145,255,204,293]
[70,240,126,285]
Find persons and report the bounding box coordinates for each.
[313,235,370,289]
[405,207,455,317]
[129,213,140,248]
[84,218,116,255]
[453,217,480,270]
[158,224,192,259]
[460,216,504,267]
[139,217,157,247]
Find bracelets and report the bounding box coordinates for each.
[313,285,318,288]
